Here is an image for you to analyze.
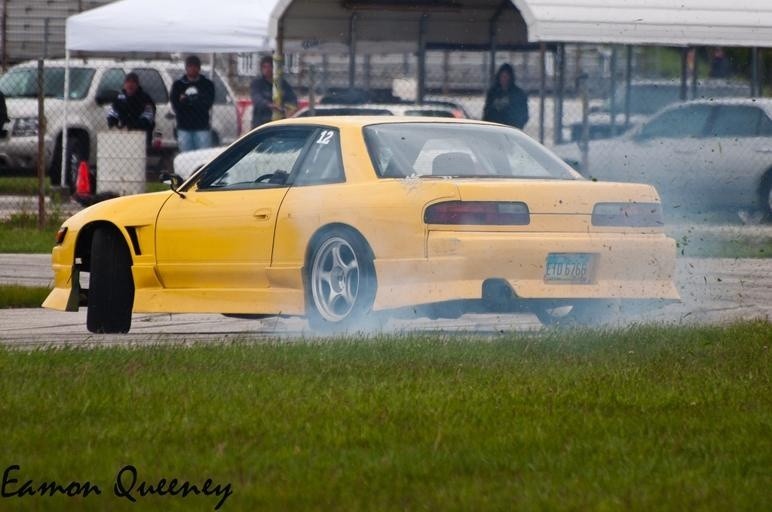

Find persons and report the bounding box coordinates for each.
[482,63,529,129]
[250,55,297,129]
[170,55,215,152]
[106,72,156,151]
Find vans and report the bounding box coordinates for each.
[0,58,239,185]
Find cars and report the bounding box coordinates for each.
[41,116,682,335]
[551,96,772,218]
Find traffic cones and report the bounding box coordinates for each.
[76,161,91,197]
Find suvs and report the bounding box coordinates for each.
[173,104,469,187]
[570,79,759,141]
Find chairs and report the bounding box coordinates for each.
[433,152,475,174]
